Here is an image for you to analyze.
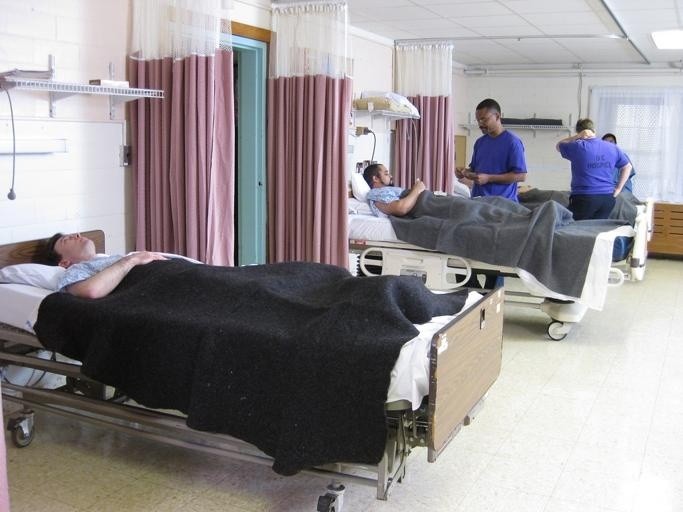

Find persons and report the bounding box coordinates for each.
[554,117,633,221]
[602,133,635,193]
[453,98,527,289]
[362,163,427,219]
[45,232,170,301]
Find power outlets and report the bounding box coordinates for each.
[357,126,368,135]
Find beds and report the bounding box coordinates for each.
[349,188,648,340]
[0,229,504,511]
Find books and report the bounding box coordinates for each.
[0,68,52,81]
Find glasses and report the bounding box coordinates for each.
[474,113,496,125]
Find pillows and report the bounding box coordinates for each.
[454,181,471,198]
[350,174,370,200]
